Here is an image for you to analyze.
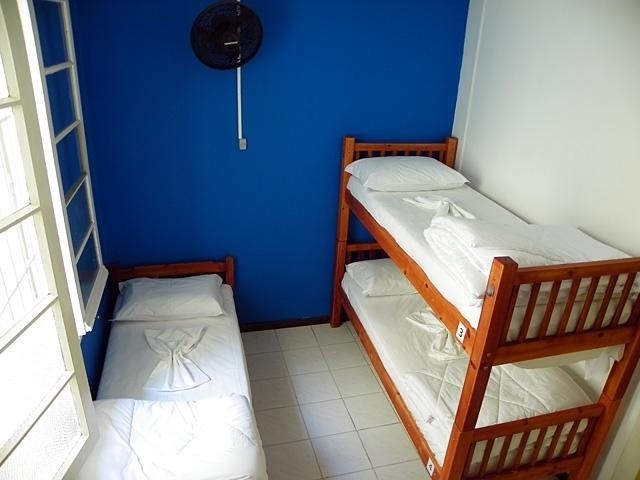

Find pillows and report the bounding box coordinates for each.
[344,155,472,192]
[106,274,225,322]
[346,258,419,297]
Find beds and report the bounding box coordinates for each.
[327,135,640,479]
[74,256,268,480]
